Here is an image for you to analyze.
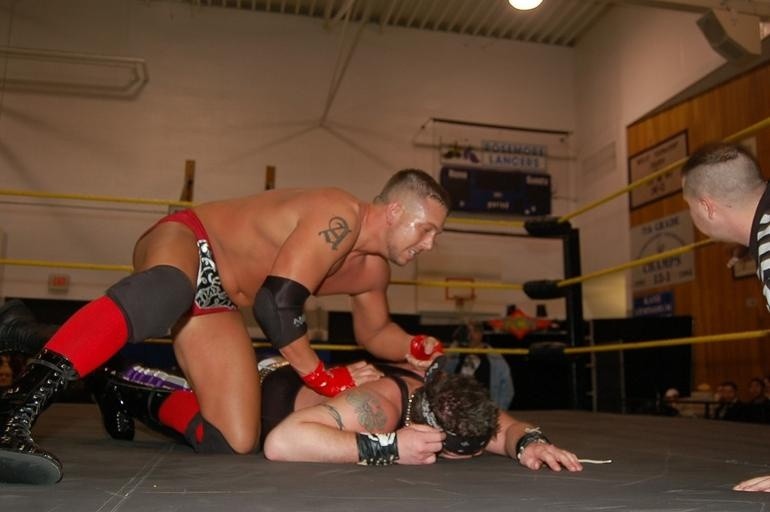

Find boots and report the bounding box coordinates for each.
[94,380,172,440]
[101,341,151,379]
[5,299,60,355]
[0,349,79,484]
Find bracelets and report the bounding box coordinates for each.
[515,426,552,461]
[354,431,400,466]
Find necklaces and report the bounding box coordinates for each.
[403,393,413,428]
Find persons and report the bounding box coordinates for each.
[0,362,14,386]
[713,380,740,419]
[680,139,770,495]
[740,376,770,425]
[1,167,450,485]
[92,345,585,473]
